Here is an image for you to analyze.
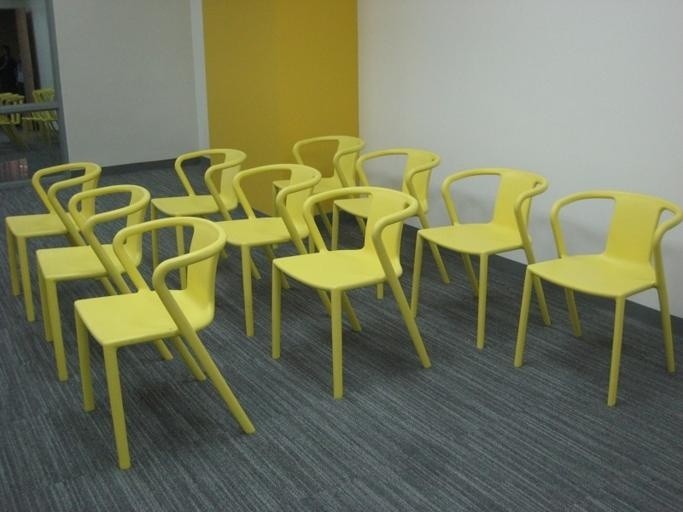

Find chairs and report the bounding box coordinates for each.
[0,87,59,150]
